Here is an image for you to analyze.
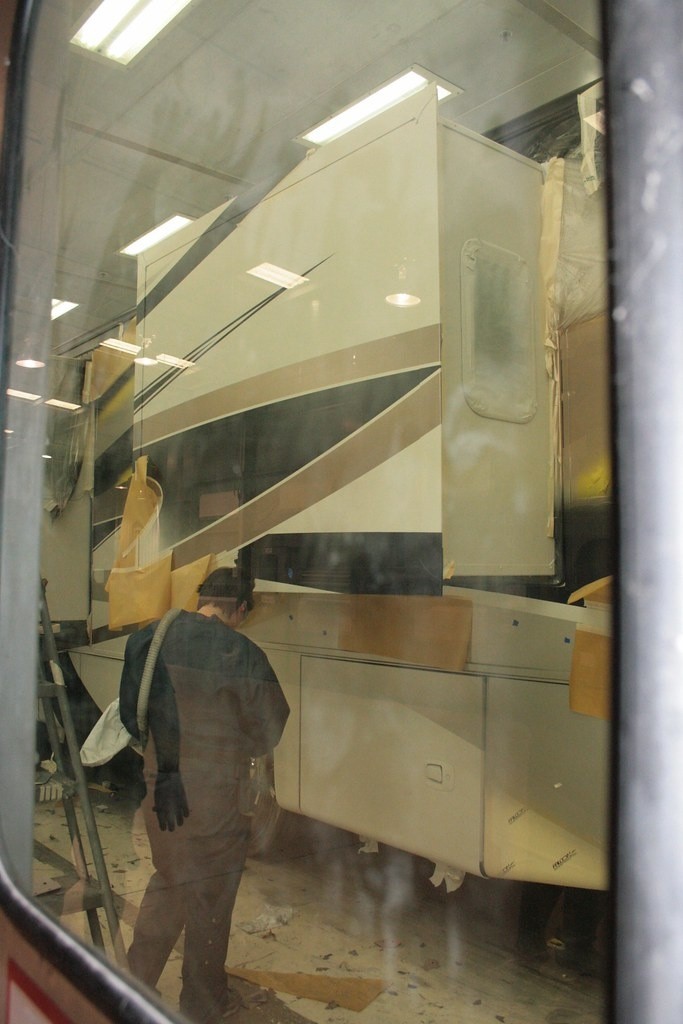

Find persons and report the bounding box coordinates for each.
[513,879,610,984]
[121,565,290,1024]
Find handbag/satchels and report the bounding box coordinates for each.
[244,748,288,859]
